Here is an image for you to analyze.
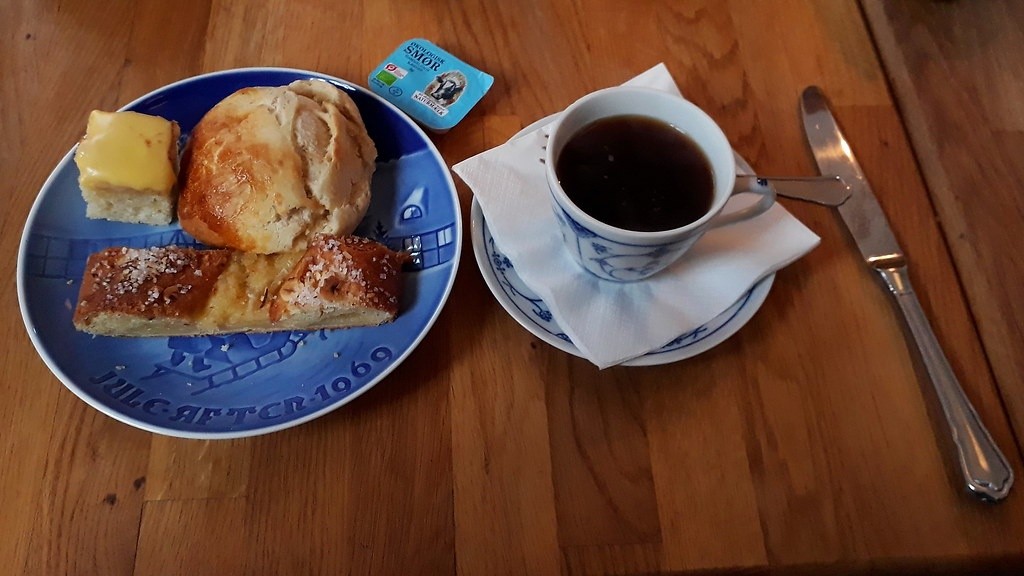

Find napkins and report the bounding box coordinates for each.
[450,61,822,370]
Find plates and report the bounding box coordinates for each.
[470,108,774,366]
[16,66,461,440]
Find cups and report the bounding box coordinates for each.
[545,86,778,283]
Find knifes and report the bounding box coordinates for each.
[798,81,1015,502]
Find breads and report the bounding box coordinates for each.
[176,80,377,252]
[73,236,414,336]
[74,109,179,226]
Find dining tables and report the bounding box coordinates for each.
[0,0,1024,576]
